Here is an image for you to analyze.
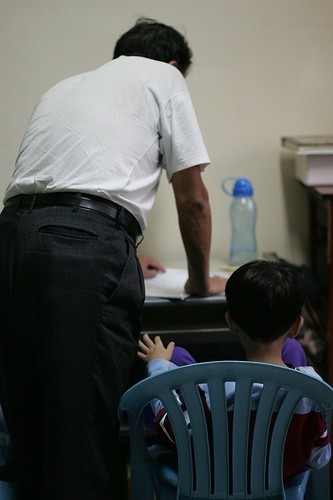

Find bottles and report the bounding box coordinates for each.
[222,176,258,265]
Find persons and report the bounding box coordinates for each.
[1,17,231,499]
[135,259,333,492]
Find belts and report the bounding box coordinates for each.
[4,192,143,243]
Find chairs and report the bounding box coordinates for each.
[117,361,333,499]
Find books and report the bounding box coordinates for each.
[280,134,333,152]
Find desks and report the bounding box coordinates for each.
[142,292,233,337]
[308,186,333,389]
